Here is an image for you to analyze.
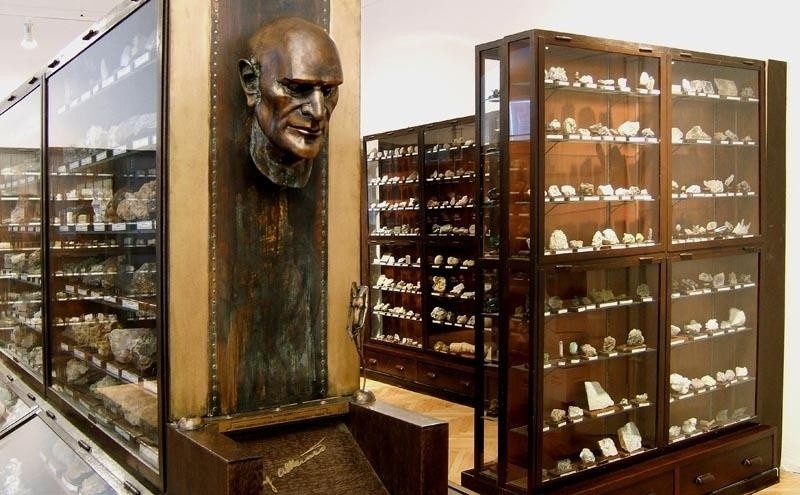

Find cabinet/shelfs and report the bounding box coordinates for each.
[428,110,478,360]
[479,98,536,369]
[664,49,769,451]
[0,364,152,495]
[503,32,664,488]
[0,70,45,396]
[473,38,505,484]
[46,0,167,490]
[358,341,498,413]
[463,425,780,495]
[360,125,427,349]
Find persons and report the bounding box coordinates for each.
[236,17,345,188]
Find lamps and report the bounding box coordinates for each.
[18,16,41,52]
